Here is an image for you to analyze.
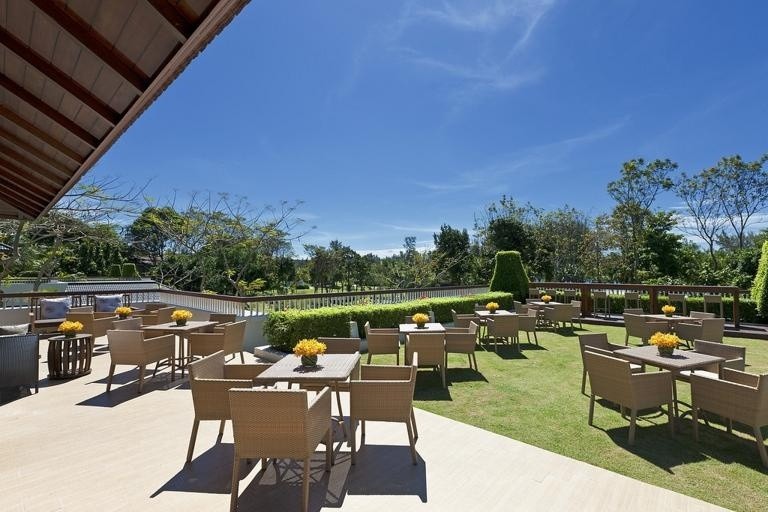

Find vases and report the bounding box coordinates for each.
[64,331,75,339]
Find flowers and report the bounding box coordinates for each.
[57,319,84,332]
[661,305,676,314]
[541,295,552,302]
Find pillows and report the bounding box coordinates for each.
[94,293,124,311]
[39,298,69,319]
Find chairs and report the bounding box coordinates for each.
[475,298,583,354]
[31,298,69,335]
[578,308,768,472]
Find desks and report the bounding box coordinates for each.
[46,333,94,380]
